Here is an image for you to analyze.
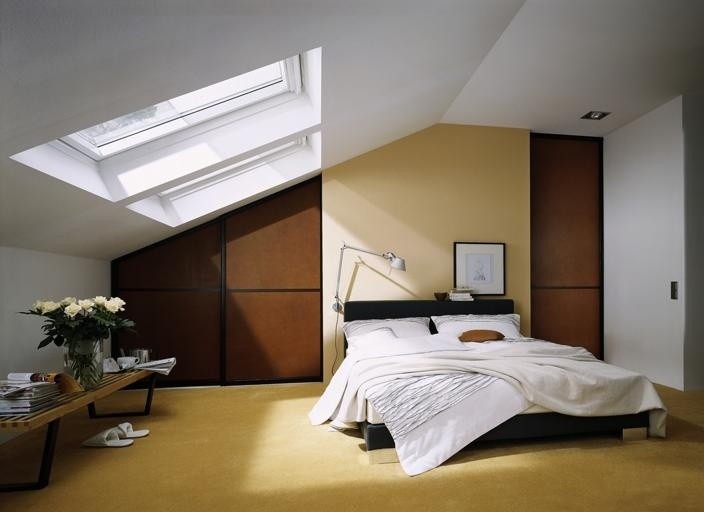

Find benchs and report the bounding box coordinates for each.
[0,362,158,492]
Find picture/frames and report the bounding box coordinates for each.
[452,241,505,297]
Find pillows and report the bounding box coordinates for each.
[458,328,505,342]
[430,313,524,339]
[341,316,431,342]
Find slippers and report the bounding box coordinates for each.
[111,422,150,439]
[80,429,134,447]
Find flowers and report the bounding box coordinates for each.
[15,295,136,350]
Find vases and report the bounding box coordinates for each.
[63,337,103,388]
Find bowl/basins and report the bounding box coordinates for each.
[433,292,448,301]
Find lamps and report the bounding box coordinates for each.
[332,243,405,314]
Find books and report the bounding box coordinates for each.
[0,371,85,415]
[448,287,475,301]
[134,357,176,376]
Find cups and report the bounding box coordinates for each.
[117,357,140,370]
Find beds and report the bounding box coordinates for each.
[341,298,650,452]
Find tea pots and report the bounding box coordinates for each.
[119,345,152,363]
